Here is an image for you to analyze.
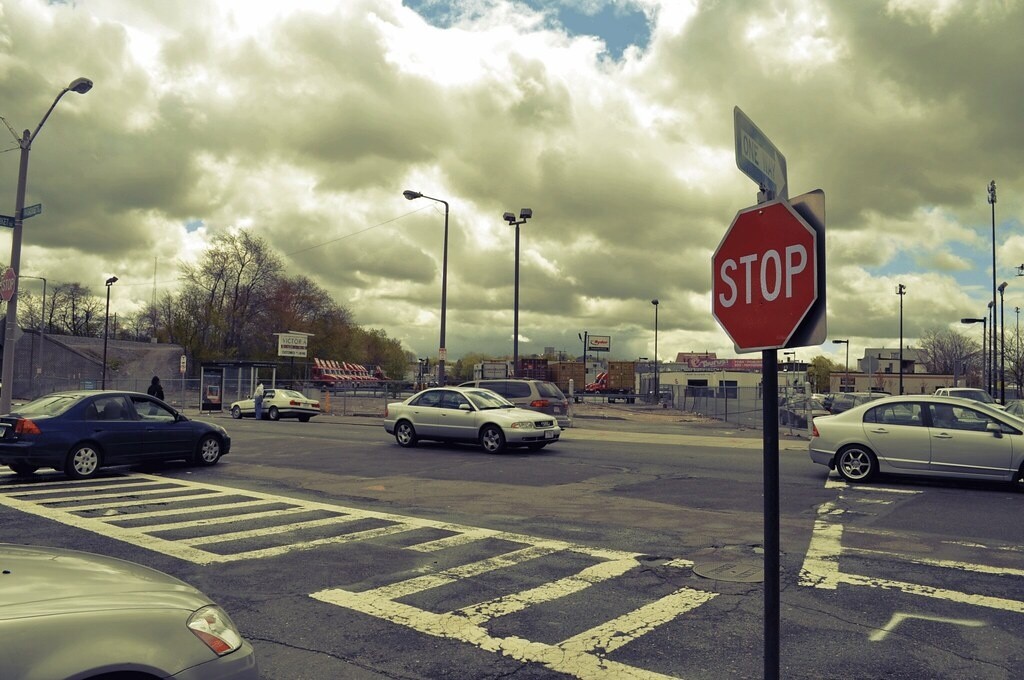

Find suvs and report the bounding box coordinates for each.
[452,378,571,429]
[824,392,838,409]
[933,387,1007,422]
[830,392,919,425]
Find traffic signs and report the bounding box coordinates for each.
[733,105,791,200]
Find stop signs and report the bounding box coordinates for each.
[0,268,16,301]
[710,197,818,352]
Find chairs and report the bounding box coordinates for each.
[104,399,123,420]
[917,406,948,427]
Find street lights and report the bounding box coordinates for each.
[403,189,450,391]
[503,207,533,375]
[651,298,659,394]
[784,351,796,384]
[0,77,95,412]
[960,282,1008,408]
[100,276,118,390]
[831,339,850,391]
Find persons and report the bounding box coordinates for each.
[147,376,165,415]
[254,380,264,420]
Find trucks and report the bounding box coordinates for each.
[310,357,391,391]
[470,357,635,395]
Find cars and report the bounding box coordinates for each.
[228,388,321,423]
[1003,403,1024,432]
[0,389,231,478]
[811,394,828,403]
[383,387,561,455]
[808,395,1024,483]
[780,398,831,427]
